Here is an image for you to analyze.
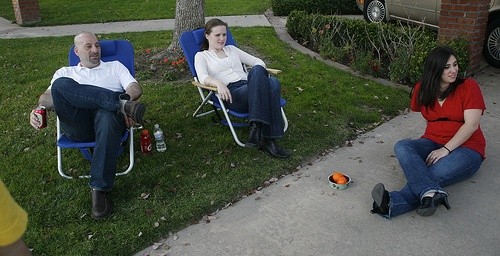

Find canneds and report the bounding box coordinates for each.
[35,105,47,129]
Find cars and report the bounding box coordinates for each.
[352,0,500,70]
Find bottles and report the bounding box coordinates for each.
[139,130,152,152]
[153,124,167,152]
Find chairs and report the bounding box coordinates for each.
[54,39,133,179]
[179,28,289,146]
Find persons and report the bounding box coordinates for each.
[193,19,291,159]
[30,32,143,220]
[0,176,34,256]
[369,46,487,220]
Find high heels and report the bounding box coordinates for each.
[418,191,451,216]
[370,183,389,215]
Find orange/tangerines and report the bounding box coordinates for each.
[332,172,347,185]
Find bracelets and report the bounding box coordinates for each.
[443,145,451,153]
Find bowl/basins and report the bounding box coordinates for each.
[327,173,351,190]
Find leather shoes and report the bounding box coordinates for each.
[123,101,145,123]
[260,138,289,159]
[245,121,261,146]
[91,189,111,219]
